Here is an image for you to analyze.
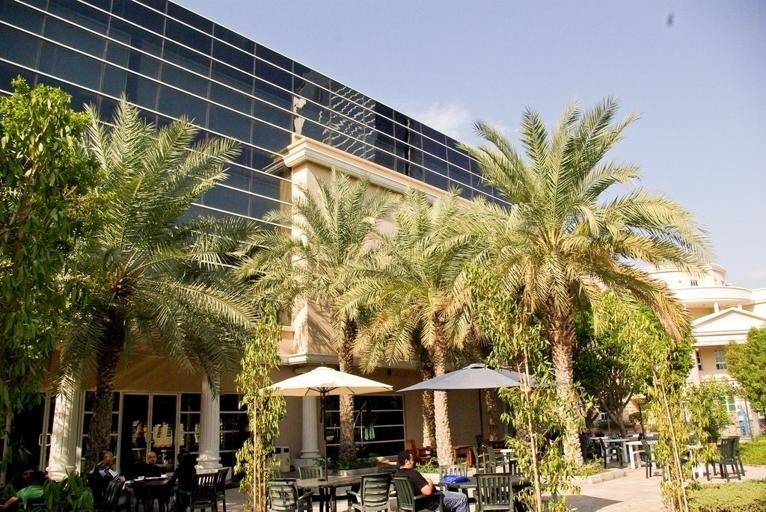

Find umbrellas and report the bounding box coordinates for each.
[395,363,557,442]
[259,363,392,512]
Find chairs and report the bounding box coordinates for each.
[0,461,232,512]
[266,465,392,511]
[590,426,746,483]
[393,439,525,511]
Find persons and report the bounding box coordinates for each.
[133,450,166,512]
[391,449,471,512]
[166,451,196,512]
[88,449,125,484]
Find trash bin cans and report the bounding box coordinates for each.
[273,446,290,473]
